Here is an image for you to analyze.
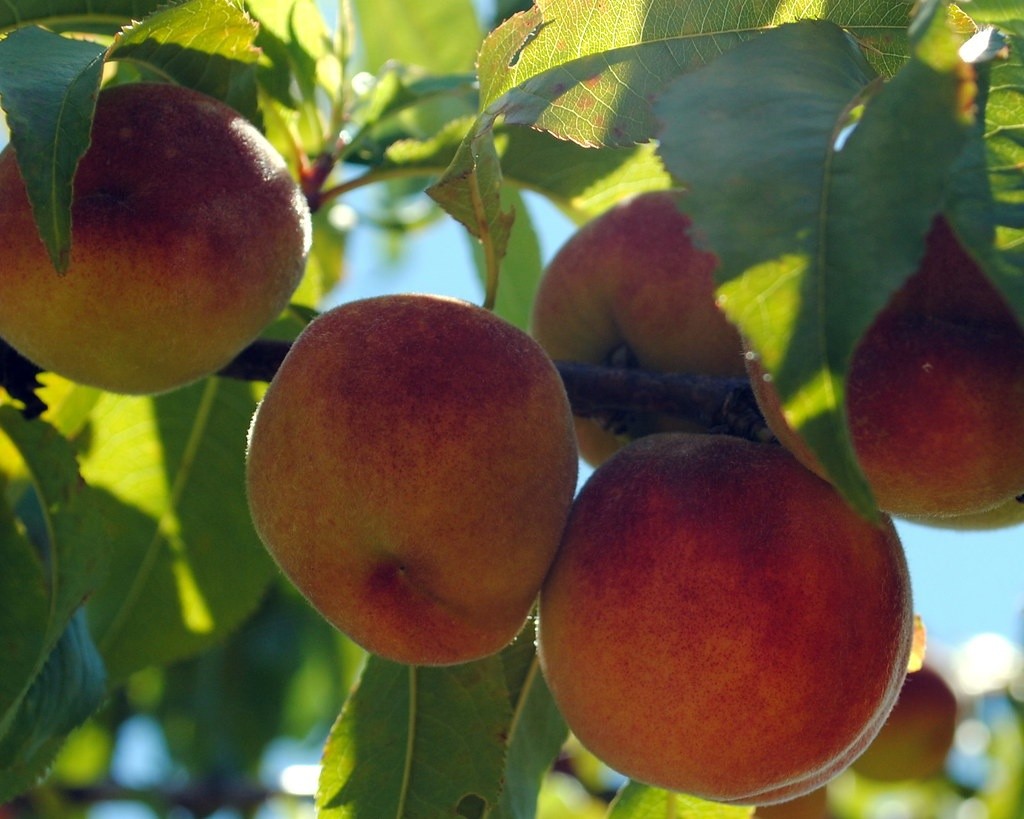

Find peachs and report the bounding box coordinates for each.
[244,187,1023,803]
[0,80,313,401]
